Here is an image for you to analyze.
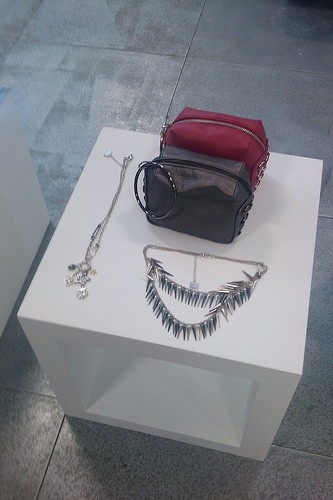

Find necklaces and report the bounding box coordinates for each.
[64,148,134,301]
[142,243,268,342]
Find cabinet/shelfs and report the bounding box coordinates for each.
[0,87,50,336]
[14,126,326,463]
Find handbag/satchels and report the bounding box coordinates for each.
[162,107,270,193]
[137,145,255,244]
